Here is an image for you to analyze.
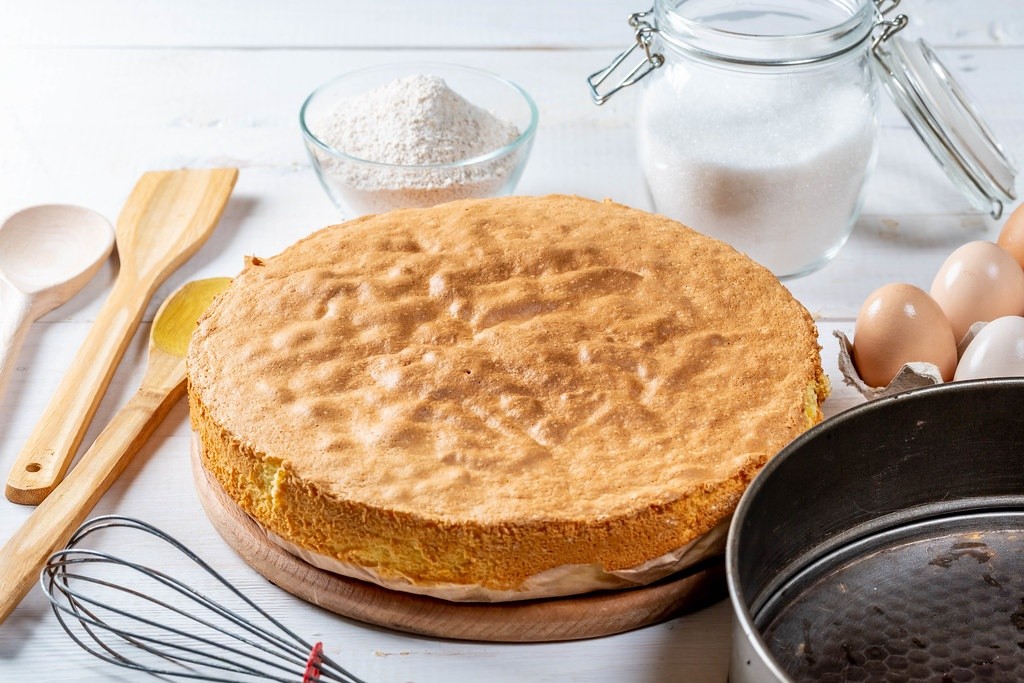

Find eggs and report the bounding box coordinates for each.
[952,316,1024,382]
[928,242,1024,343]
[996,202,1024,270]
[854,282,958,389]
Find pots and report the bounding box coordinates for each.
[726,376,1024,683]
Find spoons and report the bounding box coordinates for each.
[0,202,117,454]
[0,276,234,620]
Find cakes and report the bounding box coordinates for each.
[185,196,822,600]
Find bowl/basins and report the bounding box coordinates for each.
[295,57,538,224]
